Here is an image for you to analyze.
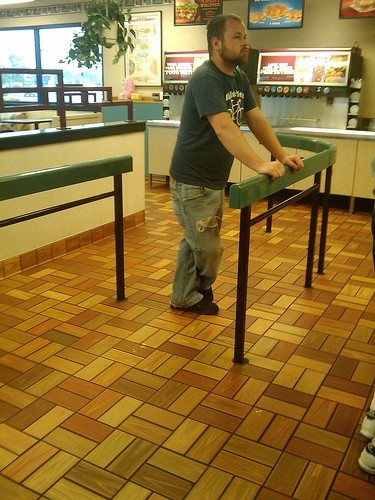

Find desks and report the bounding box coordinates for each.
[0,118,52,129]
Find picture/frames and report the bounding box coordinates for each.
[173,0,225,27]
[247,0,304,30]
[123,10,164,87]
[338,0,375,19]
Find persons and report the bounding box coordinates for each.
[168,14,303,316]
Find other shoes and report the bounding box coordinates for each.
[170,296,219,315]
[198,286,214,301]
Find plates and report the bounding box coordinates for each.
[349,4,375,13]
[264,11,289,20]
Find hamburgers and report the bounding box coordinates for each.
[249,4,302,24]
[175,0,199,24]
